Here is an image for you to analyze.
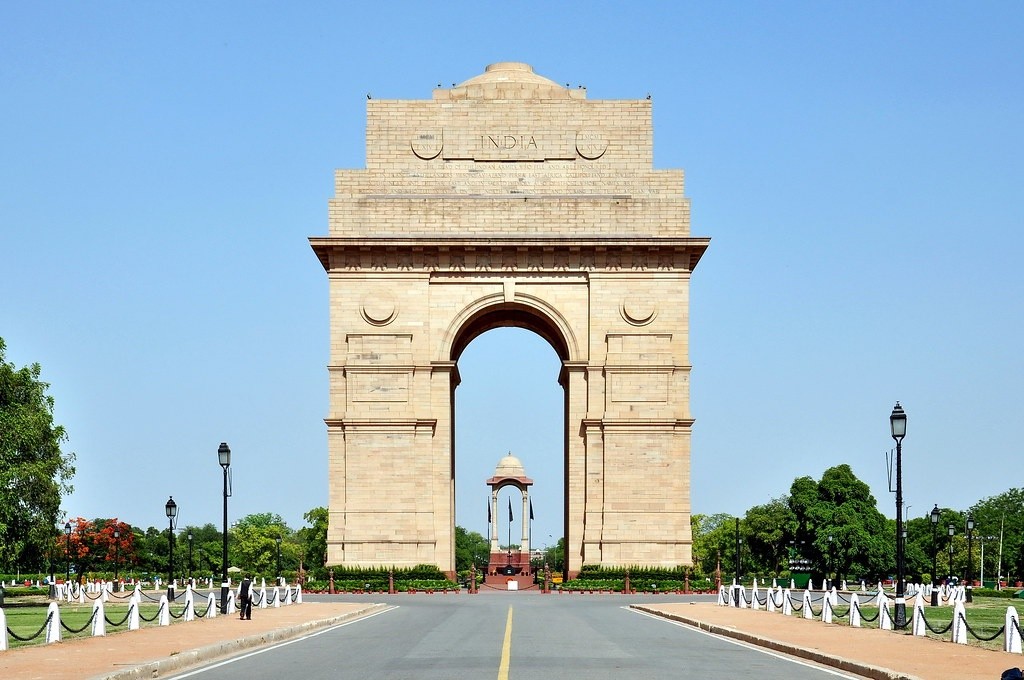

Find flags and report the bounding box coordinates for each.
[530,500,533,520]
[509,500,514,522]
[488,501,491,522]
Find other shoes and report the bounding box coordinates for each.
[240,617,245,620]
[247,617,251,620]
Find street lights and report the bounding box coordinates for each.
[889,401,907,631]
[187,530,193,586]
[966,514,974,603]
[948,516,955,601]
[275,534,282,586]
[734,518,741,607]
[217,442,232,614]
[199,543,202,588]
[165,495,177,601]
[930,504,941,606]
[114,528,119,593]
[49,525,56,600]
[65,523,72,587]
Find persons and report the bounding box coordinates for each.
[236,573,253,619]
